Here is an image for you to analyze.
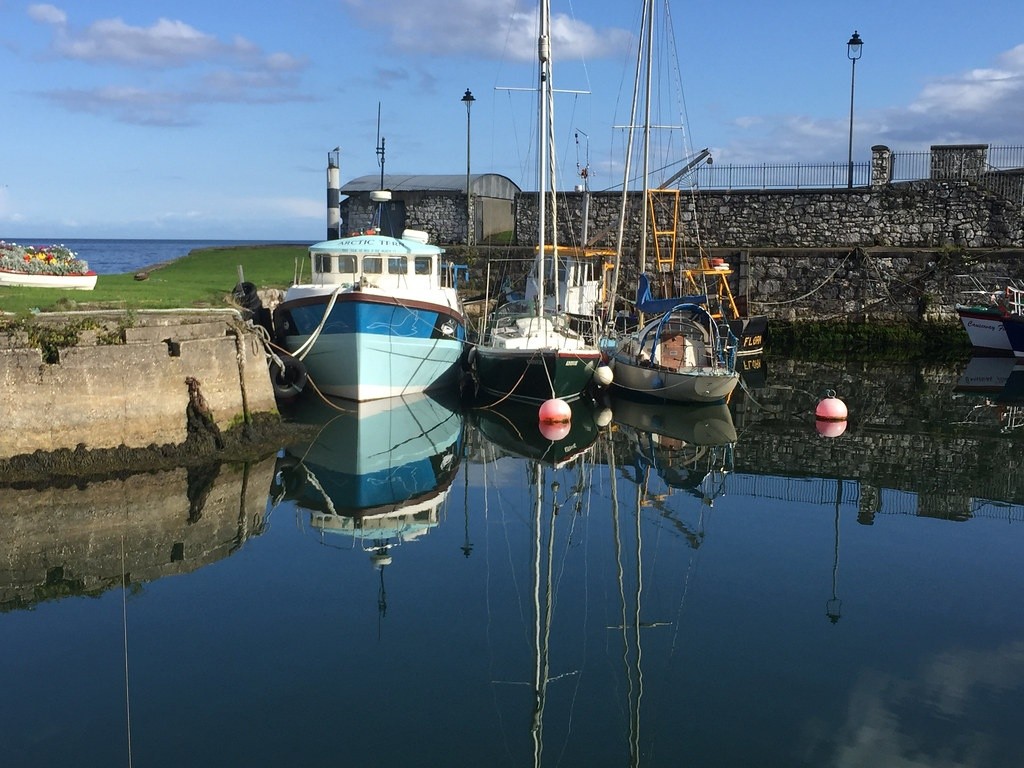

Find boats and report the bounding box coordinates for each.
[265,389,466,634]
[222,98,475,405]
[952,350,1024,435]
[955,272,1024,356]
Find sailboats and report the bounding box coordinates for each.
[457,0,762,404]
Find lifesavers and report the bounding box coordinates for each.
[709,263,730,271]
[271,458,309,502]
[346,230,377,237]
[461,345,475,371]
[269,353,307,398]
[232,282,263,312]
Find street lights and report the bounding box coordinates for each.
[844,27,864,191]
[459,87,477,245]
